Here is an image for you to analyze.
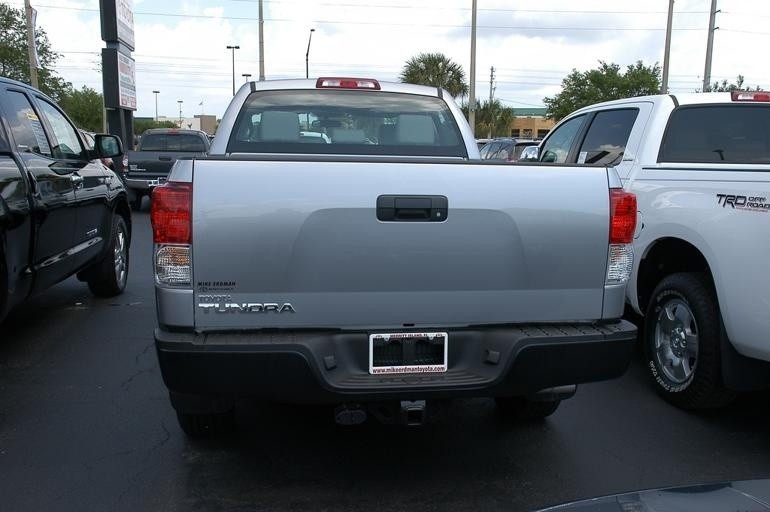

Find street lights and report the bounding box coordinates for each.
[306,29,315,78]
[242,74,251,83]
[178,101,183,127]
[227,45,240,97]
[153,91,160,122]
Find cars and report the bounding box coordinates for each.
[0,77,132,322]
[477,137,541,161]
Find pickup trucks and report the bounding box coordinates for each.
[519,91,770,406]
[123,128,211,209]
[150,77,638,442]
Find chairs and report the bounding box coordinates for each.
[258,110,437,146]
